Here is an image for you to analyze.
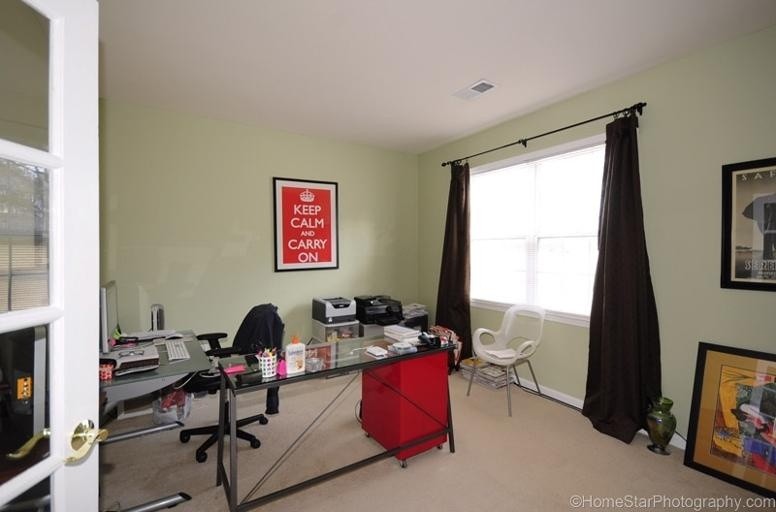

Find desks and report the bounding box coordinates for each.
[98,330,211,512]
[217,334,458,512]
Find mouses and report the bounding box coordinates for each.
[165,333,183,339]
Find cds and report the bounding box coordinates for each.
[393,342,412,350]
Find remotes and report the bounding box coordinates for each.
[115,364,159,377]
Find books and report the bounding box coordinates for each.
[459,355,515,390]
[383,325,421,343]
[402,302,427,320]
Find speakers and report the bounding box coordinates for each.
[151,304,164,331]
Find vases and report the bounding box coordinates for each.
[646,397,676,455]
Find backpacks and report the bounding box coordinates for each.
[232,303,284,355]
[255,354,278,377]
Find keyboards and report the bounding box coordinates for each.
[165,339,190,361]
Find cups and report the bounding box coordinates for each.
[307,357,322,373]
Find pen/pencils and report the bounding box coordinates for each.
[258,345,281,374]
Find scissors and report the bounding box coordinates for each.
[251,336,266,353]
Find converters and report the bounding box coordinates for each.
[368,346,388,356]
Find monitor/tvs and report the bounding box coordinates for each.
[101,280,119,354]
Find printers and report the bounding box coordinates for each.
[354,295,402,326]
[312,296,357,324]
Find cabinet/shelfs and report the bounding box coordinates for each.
[363,325,406,336]
[312,319,361,377]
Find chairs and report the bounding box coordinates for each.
[467,305,545,416]
[179,303,285,464]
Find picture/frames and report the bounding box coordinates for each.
[682,341,776,502]
[272,176,339,272]
[720,157,776,292]
